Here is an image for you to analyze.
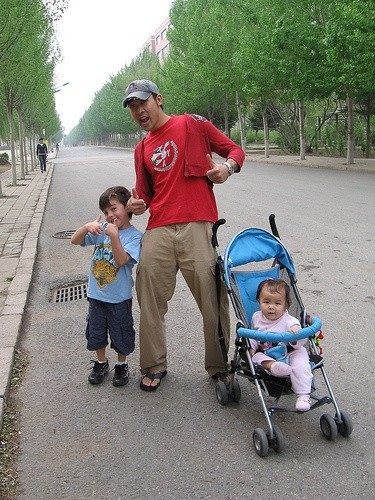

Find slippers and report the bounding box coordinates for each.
[140,370,167,391]
[213,371,237,387]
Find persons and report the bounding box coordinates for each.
[249,278,314,411]
[71,186,144,387]
[36,136,59,173]
[122,79,245,392]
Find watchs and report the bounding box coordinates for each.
[224,162,233,176]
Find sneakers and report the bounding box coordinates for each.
[87,359,110,384]
[112,364,128,386]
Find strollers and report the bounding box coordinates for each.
[210,213,355,459]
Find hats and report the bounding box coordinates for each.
[122,80,160,108]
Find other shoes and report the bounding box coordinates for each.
[295,394,311,411]
[271,361,292,376]
